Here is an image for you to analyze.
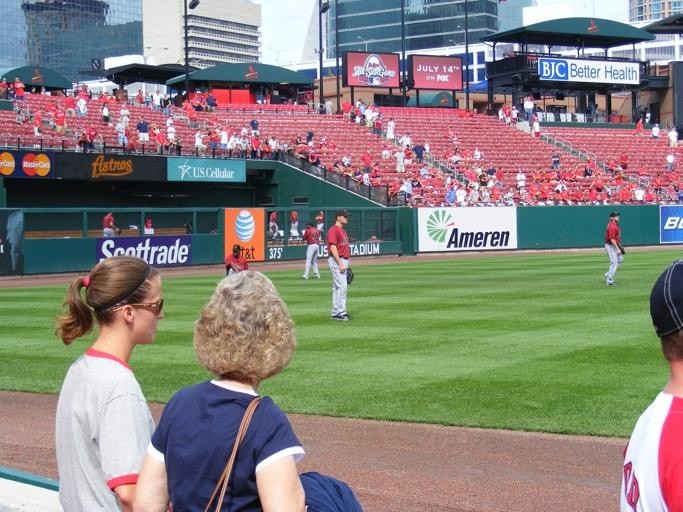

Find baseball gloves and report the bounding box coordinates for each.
[619,243,626,254]
[346,268,353,284]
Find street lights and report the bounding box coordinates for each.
[183,1,201,104]
[316,2,333,108]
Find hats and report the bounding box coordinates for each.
[648,259,682,338]
[336,208,351,217]
[610,212,620,217]
[233,245,240,251]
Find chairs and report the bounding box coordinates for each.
[0,90,277,158]
[277,102,682,207]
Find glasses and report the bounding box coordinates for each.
[112,298,164,316]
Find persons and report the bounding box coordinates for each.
[316,211,325,242]
[270,212,278,239]
[135,269,307,512]
[103,212,120,238]
[290,211,299,241]
[0,76,293,160]
[602,211,625,285]
[325,208,353,322]
[51,255,174,511]
[144,218,154,235]
[618,258,683,512]
[295,95,682,208]
[302,222,323,279]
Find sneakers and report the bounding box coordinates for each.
[337,314,352,321]
[331,315,338,319]
